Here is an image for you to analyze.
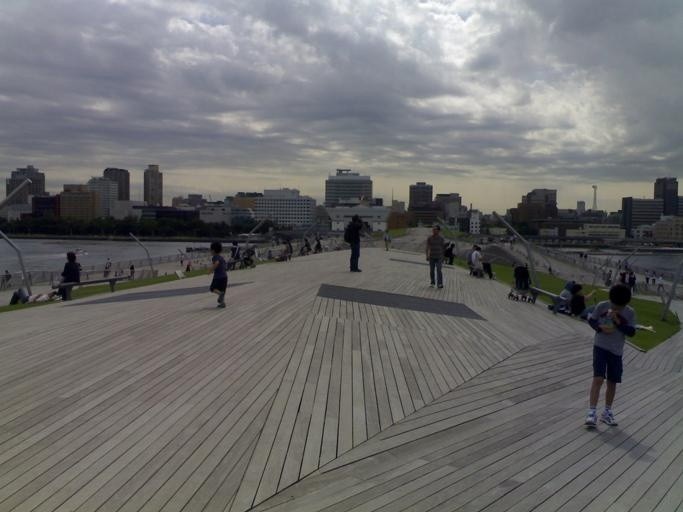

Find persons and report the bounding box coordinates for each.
[344,215,362,272]
[443,239,667,317]
[426,225,445,288]
[106,257,112,271]
[130,265,135,276]
[206,241,228,309]
[56,251,81,301]
[585,284,636,427]
[227,232,342,270]
[384,229,391,250]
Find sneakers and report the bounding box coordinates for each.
[583,414,596,428]
[599,410,617,426]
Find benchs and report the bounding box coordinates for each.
[49,236,325,305]
[420,246,569,318]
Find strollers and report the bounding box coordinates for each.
[503,264,540,304]
[239,244,257,268]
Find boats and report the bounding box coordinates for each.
[73,245,89,259]
